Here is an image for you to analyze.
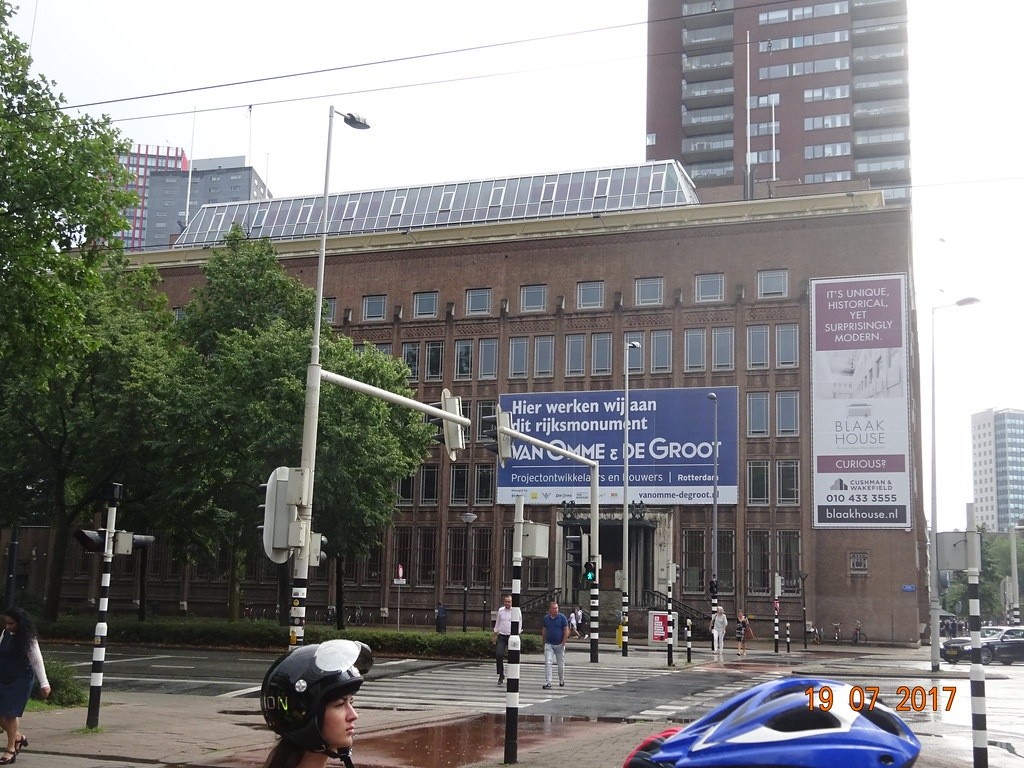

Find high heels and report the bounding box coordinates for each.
[15,736,28,756]
[0,749,16,765]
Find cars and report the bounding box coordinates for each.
[940,625,1024,666]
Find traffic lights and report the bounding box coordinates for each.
[667,562,681,584]
[253,465,294,565]
[709,579,719,595]
[564,525,589,584]
[113,532,155,555]
[73,528,107,553]
[310,531,329,567]
[939,570,951,589]
[481,404,514,469]
[583,561,599,585]
[428,388,466,462]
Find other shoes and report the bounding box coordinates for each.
[543,684,551,689]
[584,634,588,640]
[498,675,505,685]
[578,636,582,640]
[737,652,740,656]
[743,653,746,656]
[712,650,716,654]
[572,635,579,638]
[719,652,722,654]
[560,677,564,687]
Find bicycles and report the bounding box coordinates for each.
[810,622,826,645]
[830,622,843,646]
[347,604,368,628]
[851,617,869,647]
[321,603,336,626]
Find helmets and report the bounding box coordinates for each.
[260,644,364,752]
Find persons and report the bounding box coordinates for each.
[940,617,965,639]
[542,602,569,689]
[260,638,374,768]
[437,603,446,616]
[707,606,727,655]
[0,608,53,766]
[736,609,749,656]
[492,594,523,685]
[567,606,588,640]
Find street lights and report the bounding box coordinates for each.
[928,295,981,673]
[706,390,719,650]
[480,567,493,631]
[286,103,373,654]
[457,512,477,632]
[798,572,808,649]
[621,340,644,658]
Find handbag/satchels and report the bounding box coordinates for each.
[744,622,754,639]
[711,614,717,629]
